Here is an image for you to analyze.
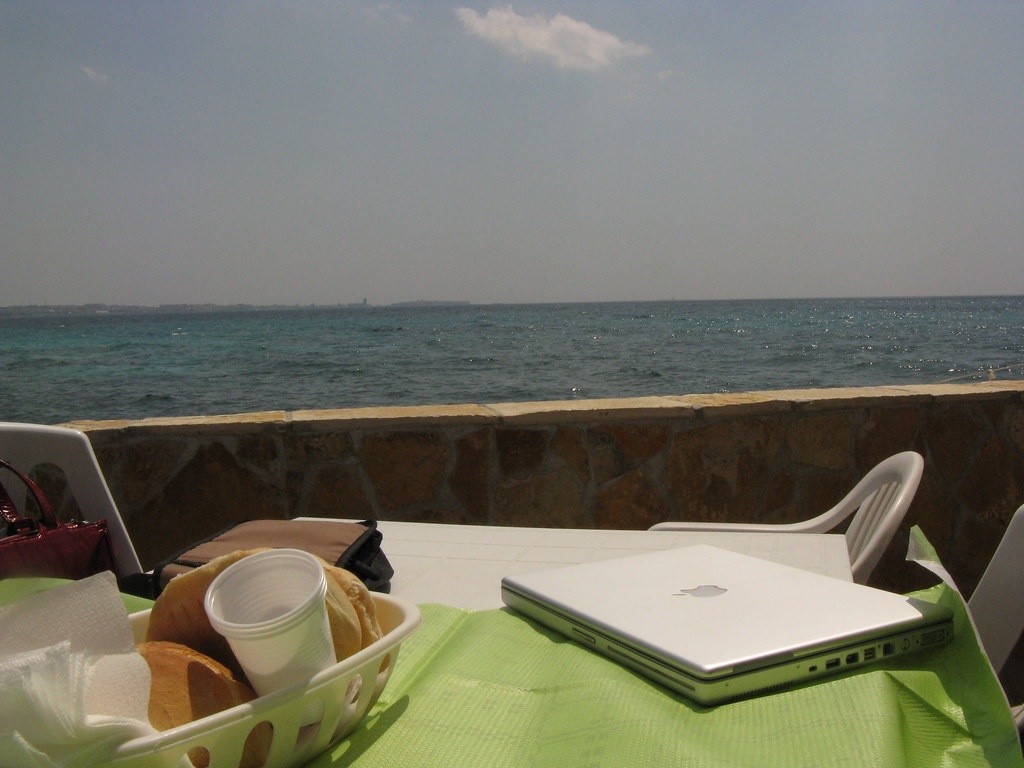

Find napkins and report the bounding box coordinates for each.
[0,571,195,768]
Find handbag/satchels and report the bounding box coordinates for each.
[0,458,119,580]
[152,519,394,594]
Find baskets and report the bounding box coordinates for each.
[0,590,421,768]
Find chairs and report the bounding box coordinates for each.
[0,422,144,577]
[649,451,924,586]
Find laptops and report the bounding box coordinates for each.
[501,543,955,706]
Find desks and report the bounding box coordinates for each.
[0,517,1024,768]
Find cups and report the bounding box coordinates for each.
[203,548,338,727]
